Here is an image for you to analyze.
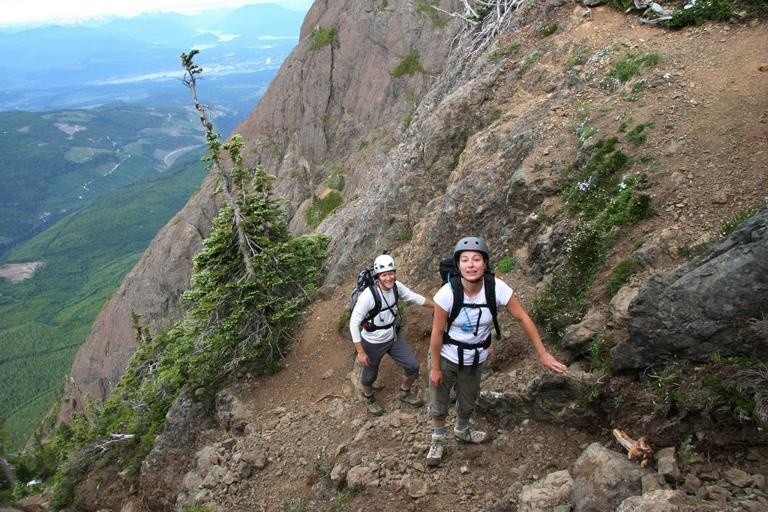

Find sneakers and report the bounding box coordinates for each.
[362,393,383,416]
[454,425,490,444]
[399,387,423,408]
[426,434,444,466]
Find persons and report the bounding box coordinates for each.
[349,255,436,417]
[425,237,568,467]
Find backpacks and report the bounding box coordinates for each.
[439,256,501,341]
[349,266,398,321]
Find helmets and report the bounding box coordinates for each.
[453,237,489,261]
[372,255,397,276]
